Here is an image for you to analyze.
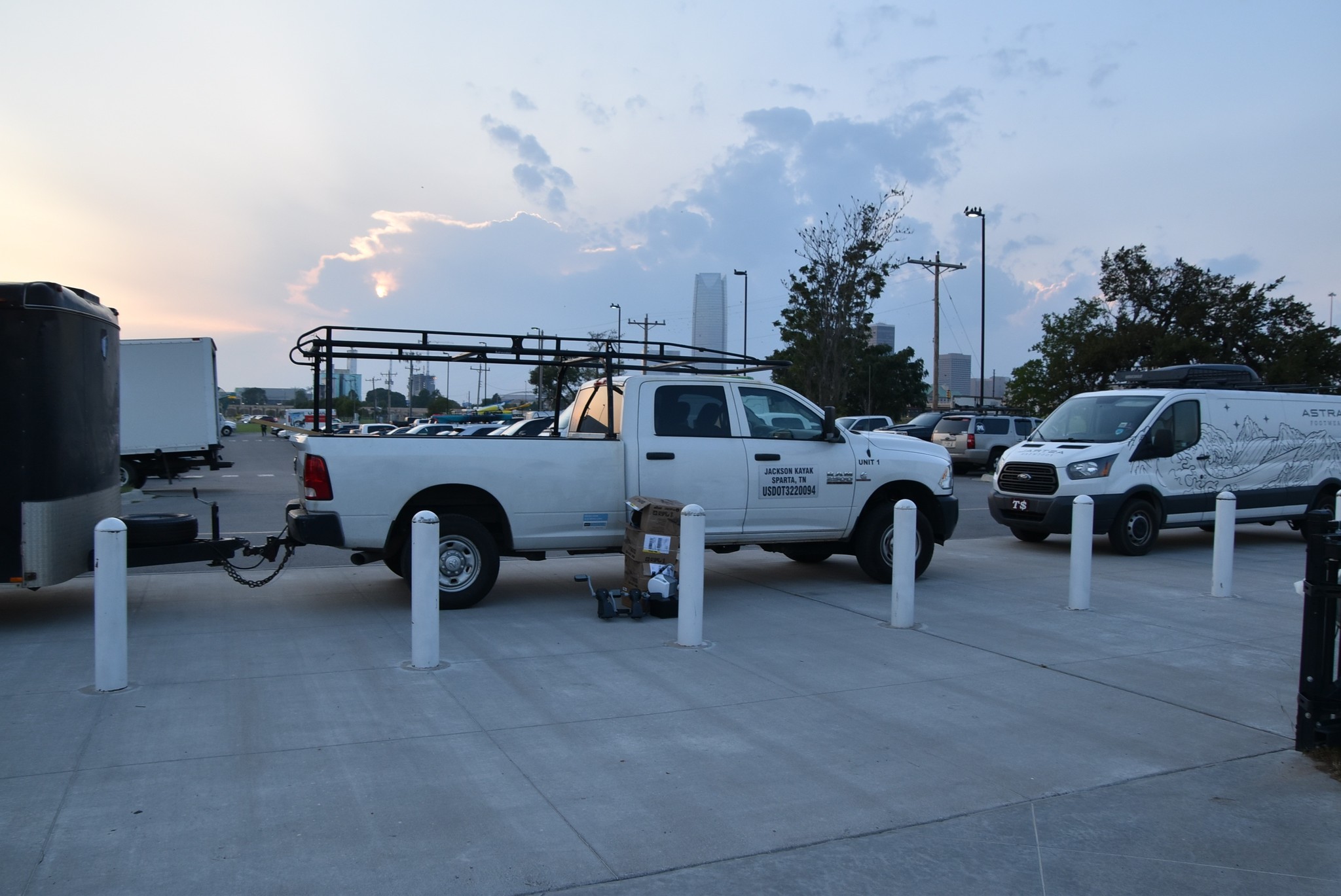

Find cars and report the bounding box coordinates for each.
[236,414,279,425]
[270,423,292,438]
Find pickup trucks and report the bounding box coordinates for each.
[284,373,960,612]
[834,415,895,433]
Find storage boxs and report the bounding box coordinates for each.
[618,496,685,608]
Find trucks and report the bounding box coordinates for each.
[219,413,237,436]
[284,408,343,433]
[119,337,234,489]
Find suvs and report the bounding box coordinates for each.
[871,405,1059,476]
[315,414,563,438]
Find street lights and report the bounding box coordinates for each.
[479,341,487,407]
[531,327,541,411]
[963,207,987,405]
[443,352,449,414]
[733,269,748,377]
[609,303,621,376]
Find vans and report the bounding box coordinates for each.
[755,412,813,432]
[988,364,1341,555]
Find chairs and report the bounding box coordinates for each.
[693,403,725,436]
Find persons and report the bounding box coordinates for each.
[261,424,267,436]
[695,402,721,435]
